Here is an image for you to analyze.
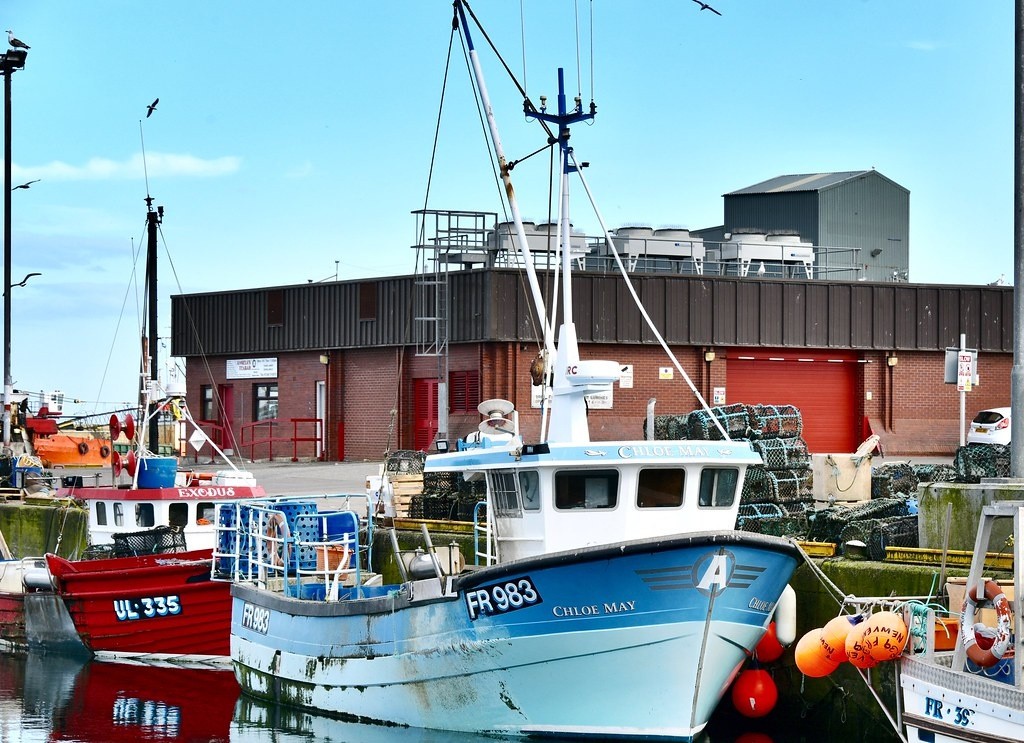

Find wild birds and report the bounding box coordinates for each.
[694,0,722,16]
[2,272,42,297]
[146,97,159,118]
[12,179,42,191]
[5,29,31,52]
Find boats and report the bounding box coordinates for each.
[45,662,240,741]
[900,501,1018,743]
[1,99,262,662]
[231,0,801,740]
[1,28,131,502]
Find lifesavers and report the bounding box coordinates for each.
[264,512,292,568]
[99,445,111,458]
[185,472,217,481]
[958,579,1013,669]
[78,442,89,455]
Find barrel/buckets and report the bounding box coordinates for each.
[132,457,177,489]
[316,510,360,568]
[932,618,962,651]
[285,584,402,601]
[364,474,395,518]
[844,544,868,560]
[314,538,355,581]
[12,466,42,487]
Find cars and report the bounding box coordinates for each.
[967,407,1012,446]
